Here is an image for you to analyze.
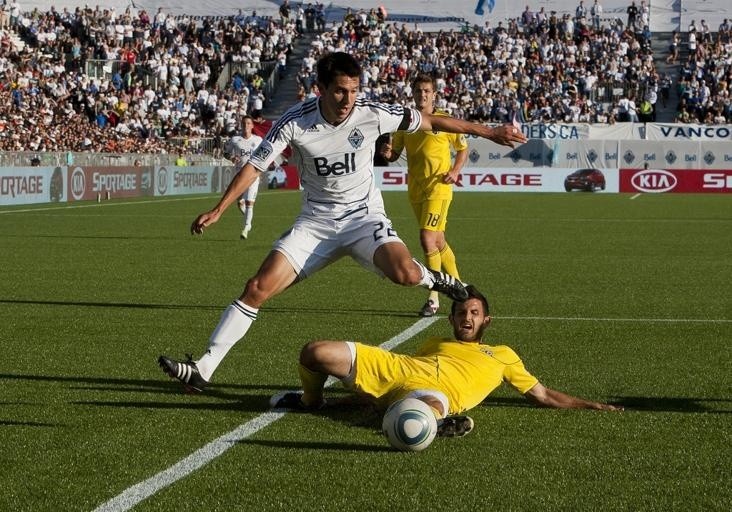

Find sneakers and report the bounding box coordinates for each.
[269,391,327,413]
[419,299,441,318]
[240,222,253,240]
[436,414,473,437]
[159,354,210,393]
[425,269,468,304]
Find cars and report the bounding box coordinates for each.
[141,167,152,193]
[265,168,288,188]
[564,168,606,192]
[49,167,64,201]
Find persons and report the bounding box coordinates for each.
[269,283,627,440]
[222,115,265,241]
[1,0,732,168]
[380,74,470,318]
[158,53,530,395]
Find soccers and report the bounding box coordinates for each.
[382,398,437,452]
